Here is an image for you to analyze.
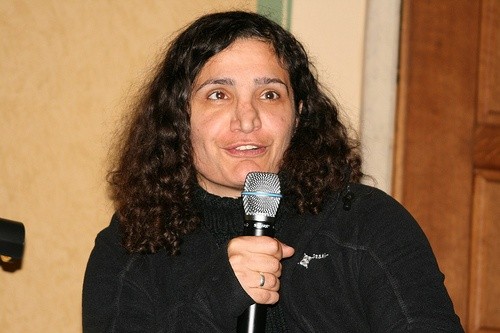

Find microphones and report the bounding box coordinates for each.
[237,171,282,333]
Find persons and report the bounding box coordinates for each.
[81,12,468,333]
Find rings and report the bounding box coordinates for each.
[259,273,265,286]
